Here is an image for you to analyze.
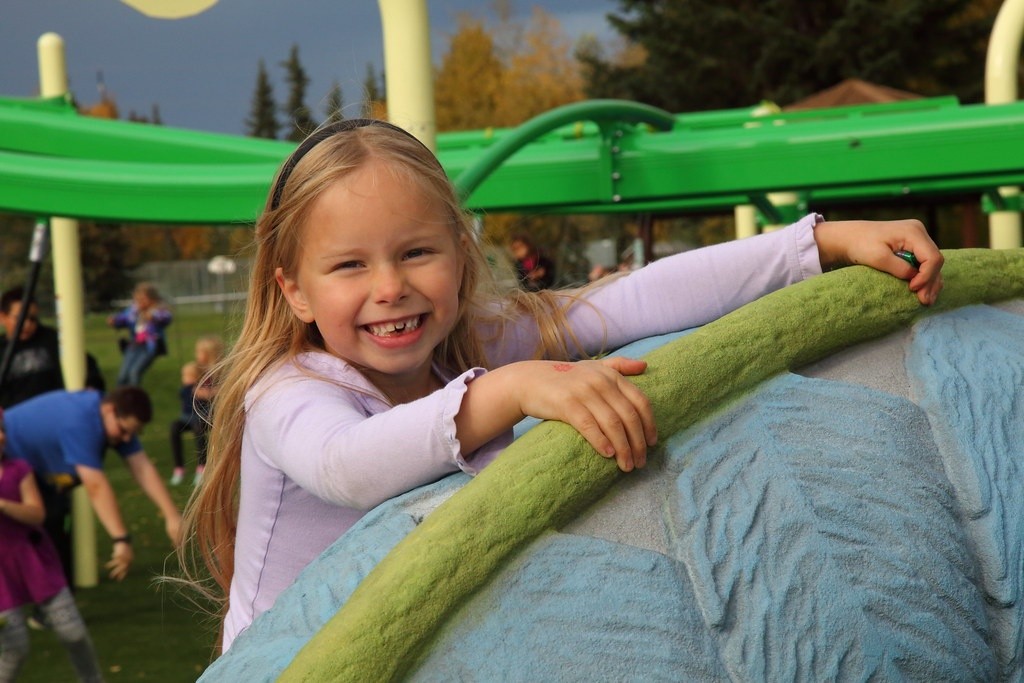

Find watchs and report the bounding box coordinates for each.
[113,535,131,542]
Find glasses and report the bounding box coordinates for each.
[27,314,37,321]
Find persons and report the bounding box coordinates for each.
[0,284,64,408]
[171,338,224,487]
[1,409,104,683]
[4,385,182,631]
[513,237,556,292]
[177,118,946,654]
[108,286,173,390]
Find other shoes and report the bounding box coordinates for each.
[193,473,201,487]
[27,608,48,630]
[170,472,183,486]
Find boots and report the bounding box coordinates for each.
[63,634,103,683]
